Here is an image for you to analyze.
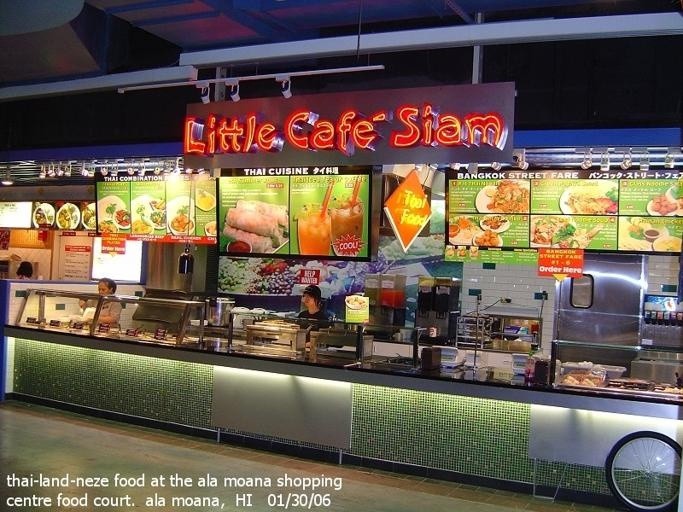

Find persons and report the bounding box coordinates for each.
[15,261,33,281]
[77,277,121,330]
[294,285,333,348]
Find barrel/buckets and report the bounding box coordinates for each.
[205,295,236,327]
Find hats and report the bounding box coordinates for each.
[300,285,321,297]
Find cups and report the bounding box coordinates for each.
[297,208,332,256]
[328,191,364,257]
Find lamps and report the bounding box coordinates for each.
[194,74,297,105]
[581,147,674,171]
[2,165,14,186]
[39,155,206,180]
[178,173,194,273]
[415,148,529,175]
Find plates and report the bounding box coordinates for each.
[479,214,510,233]
[526,210,582,250]
[449,212,480,245]
[646,197,680,216]
[475,182,529,213]
[558,179,618,215]
[665,184,683,216]
[617,214,683,252]
[472,231,503,247]
[31,195,218,236]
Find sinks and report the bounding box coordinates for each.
[381,356,422,367]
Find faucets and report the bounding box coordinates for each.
[412,326,427,369]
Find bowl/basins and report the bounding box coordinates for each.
[344,294,367,310]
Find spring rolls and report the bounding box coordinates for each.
[222,200,289,254]
